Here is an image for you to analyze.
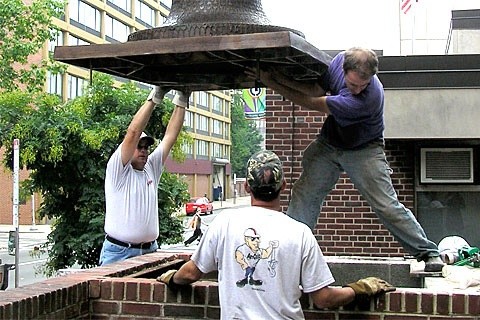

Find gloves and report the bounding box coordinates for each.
[157,269,178,286]
[342,277,395,300]
[172,90,192,108]
[147,86,168,103]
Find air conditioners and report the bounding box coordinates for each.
[421,148,473,183]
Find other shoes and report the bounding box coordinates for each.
[424,257,444,272]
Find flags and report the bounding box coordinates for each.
[401,0,412,14]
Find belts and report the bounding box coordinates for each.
[106,235,155,249]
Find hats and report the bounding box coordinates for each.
[246,149,284,195]
[137,131,155,147]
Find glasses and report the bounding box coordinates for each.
[138,144,150,151]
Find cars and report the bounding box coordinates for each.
[185,196,214,215]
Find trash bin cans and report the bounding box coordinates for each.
[8,231,15,254]
[214,188,219,199]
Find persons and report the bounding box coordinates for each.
[184,207,208,247]
[99,85,191,266]
[245,45,444,272]
[157,149,395,320]
